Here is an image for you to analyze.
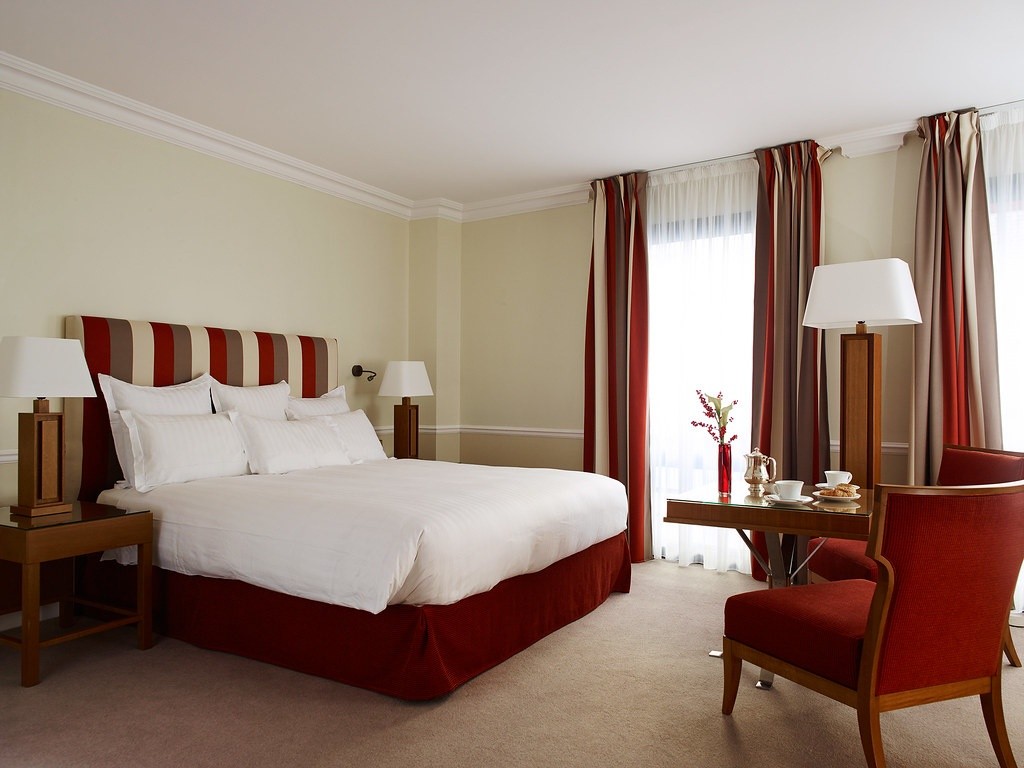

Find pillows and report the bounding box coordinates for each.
[209,375,291,421]
[227,410,355,475]
[96,371,213,489]
[286,385,351,421]
[118,409,251,492]
[283,408,396,464]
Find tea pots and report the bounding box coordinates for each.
[743,446,776,497]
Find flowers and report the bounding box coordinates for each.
[690,389,739,482]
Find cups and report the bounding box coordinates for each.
[774,480,804,500]
[824,471,852,487]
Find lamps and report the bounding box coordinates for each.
[377,361,435,459]
[802,257,923,488]
[0,335,97,518]
[352,364,377,381]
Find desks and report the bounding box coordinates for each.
[660,485,877,691]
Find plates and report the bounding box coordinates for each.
[812,491,861,502]
[764,496,814,505]
[814,482,860,491]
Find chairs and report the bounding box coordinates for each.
[807,445,1024,667]
[722,479,1024,768]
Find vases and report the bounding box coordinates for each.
[717,444,731,496]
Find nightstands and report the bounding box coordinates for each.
[0,498,154,688]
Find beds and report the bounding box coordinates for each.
[61,315,632,705]
